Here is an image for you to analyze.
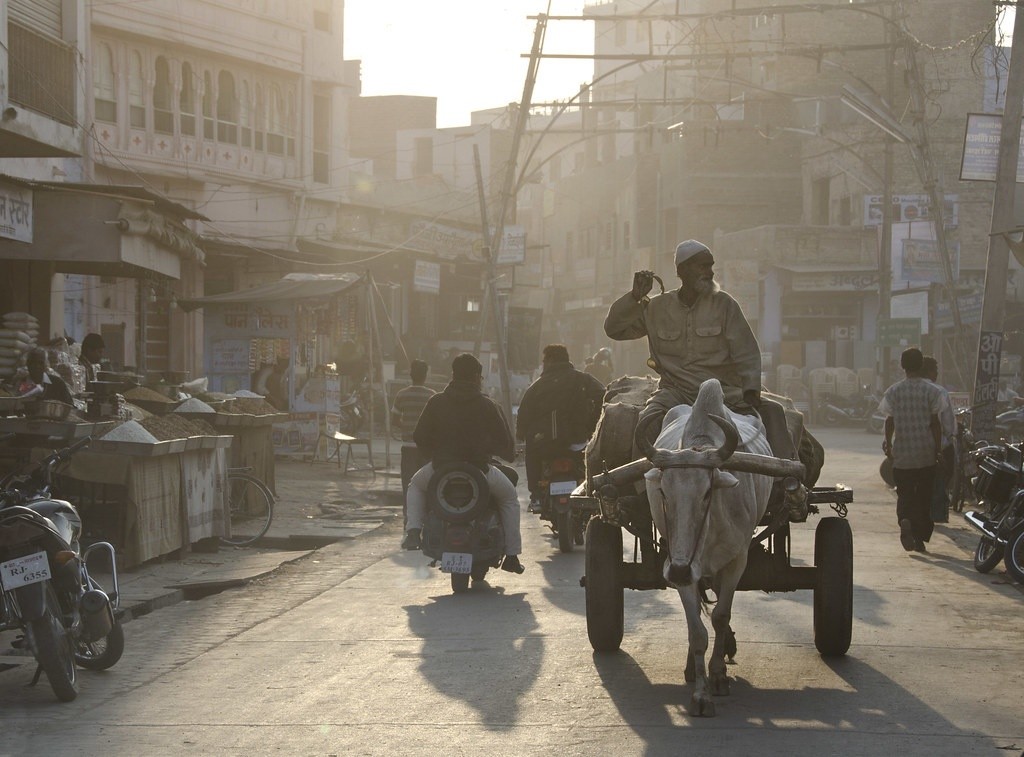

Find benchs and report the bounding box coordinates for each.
[310,429,376,478]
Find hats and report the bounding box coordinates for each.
[675,240,706,267]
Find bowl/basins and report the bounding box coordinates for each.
[22,401,73,421]
[89,369,265,412]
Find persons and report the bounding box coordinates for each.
[402,353,525,574]
[76,333,111,388]
[604,239,800,510]
[873,348,956,551]
[432,347,459,373]
[516,344,615,545]
[391,359,437,529]
[998,379,1018,401]
[251,357,325,409]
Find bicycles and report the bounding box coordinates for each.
[212,465,277,547]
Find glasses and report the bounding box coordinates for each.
[477,375,484,381]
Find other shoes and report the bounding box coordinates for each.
[571,508,578,518]
[530,488,544,502]
[621,487,663,508]
[929,514,950,524]
[769,481,787,508]
[899,517,925,553]
[502,558,525,575]
[400,537,421,548]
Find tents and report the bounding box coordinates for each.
[177,273,376,462]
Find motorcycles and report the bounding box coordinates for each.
[866,407,889,435]
[0,435,124,702]
[996,397,1024,444]
[527,458,586,552]
[817,383,881,427]
[419,446,510,594]
[964,442,1024,585]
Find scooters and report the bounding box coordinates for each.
[945,401,989,510]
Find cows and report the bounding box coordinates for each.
[633,378,775,717]
